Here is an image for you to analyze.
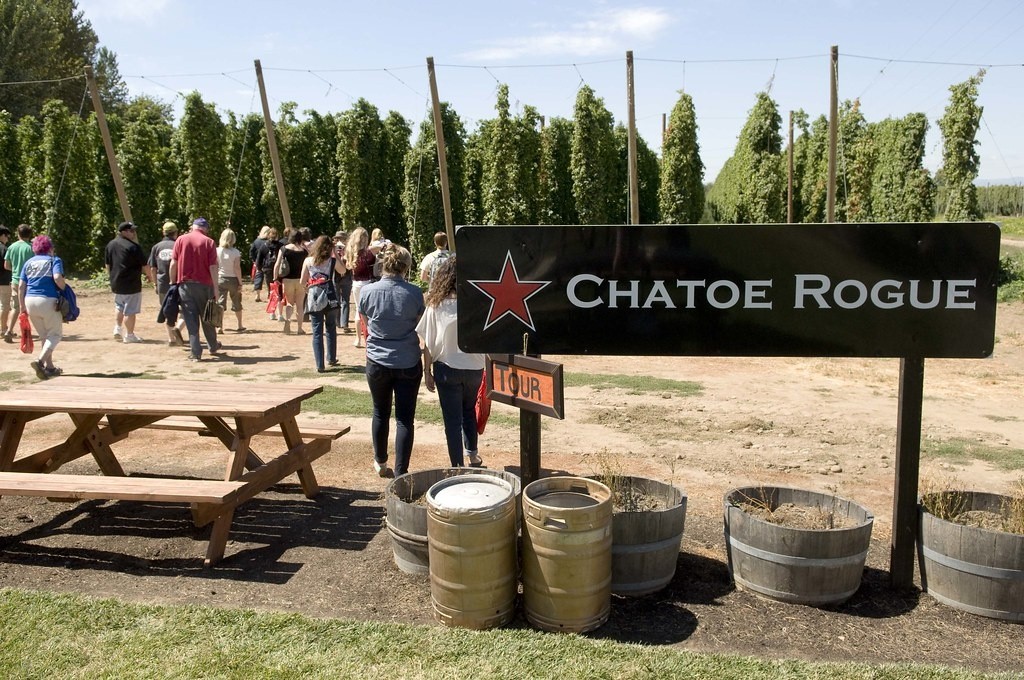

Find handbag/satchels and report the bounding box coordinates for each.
[278,246,290,277]
[18,312,34,353]
[304,280,341,315]
[475,371,491,435]
[203,297,223,327]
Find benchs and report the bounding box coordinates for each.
[98,414,351,439]
[0,471,251,504]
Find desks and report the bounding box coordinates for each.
[0,377,331,568]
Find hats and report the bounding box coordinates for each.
[193,218,208,228]
[332,231,348,239]
[163,222,178,232]
[118,221,138,232]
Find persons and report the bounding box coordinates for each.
[251,225,392,347]
[358,244,427,478]
[3,224,36,344]
[300,236,346,373]
[168,218,222,359]
[419,231,449,282]
[414,257,486,467]
[0,225,17,339]
[215,228,246,333]
[147,221,186,346]
[104,222,151,343]
[18,235,65,380]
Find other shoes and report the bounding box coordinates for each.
[318,367,325,372]
[165,324,247,362]
[42,364,61,376]
[256,295,306,334]
[0,331,17,343]
[123,335,143,342]
[113,326,123,339]
[374,459,387,475]
[468,455,482,467]
[326,359,339,365]
[30,359,49,380]
[343,328,351,333]
[354,341,361,347]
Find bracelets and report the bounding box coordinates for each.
[424,369,431,372]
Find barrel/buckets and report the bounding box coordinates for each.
[522,475,612,634]
[427,473,517,632]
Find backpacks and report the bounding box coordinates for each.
[428,251,453,291]
[261,243,277,274]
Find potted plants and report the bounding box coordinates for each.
[724,470,873,605]
[918,474,1024,622]
[580,455,686,597]
[383,464,522,576]
